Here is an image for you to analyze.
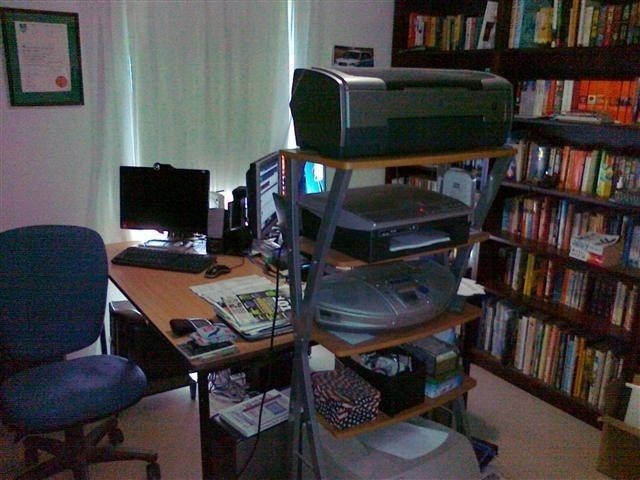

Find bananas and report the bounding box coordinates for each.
[111,247,217,273]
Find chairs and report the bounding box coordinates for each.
[0,224,161,480]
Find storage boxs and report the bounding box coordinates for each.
[595,373,640,480]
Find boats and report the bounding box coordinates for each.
[229,202,240,231]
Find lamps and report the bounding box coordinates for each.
[0,224,161,480]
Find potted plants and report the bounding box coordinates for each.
[0,6,85,108]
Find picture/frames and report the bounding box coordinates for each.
[103,236,370,480]
[0,6,85,108]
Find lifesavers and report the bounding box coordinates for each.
[109,301,190,397]
[210,388,302,480]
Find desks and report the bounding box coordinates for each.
[103,236,370,480]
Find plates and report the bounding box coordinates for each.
[279,143,520,480]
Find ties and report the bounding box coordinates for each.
[283,149,326,193]
[246,151,282,240]
[119,165,210,252]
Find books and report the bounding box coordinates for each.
[174,325,246,360]
[503,136,638,208]
[595,373,640,480]
[394,158,495,194]
[501,195,639,269]
[507,1,638,49]
[499,246,639,332]
[189,274,296,336]
[476,297,628,412]
[407,1,498,50]
[220,387,302,437]
[515,79,639,126]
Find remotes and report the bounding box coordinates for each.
[204,265,231,278]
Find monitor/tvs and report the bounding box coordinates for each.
[189,274,296,336]
[220,387,302,437]
[174,325,246,360]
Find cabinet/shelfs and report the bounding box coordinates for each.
[384,0,640,432]
[279,143,520,480]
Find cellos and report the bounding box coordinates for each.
[297,184,473,262]
[288,66,515,158]
